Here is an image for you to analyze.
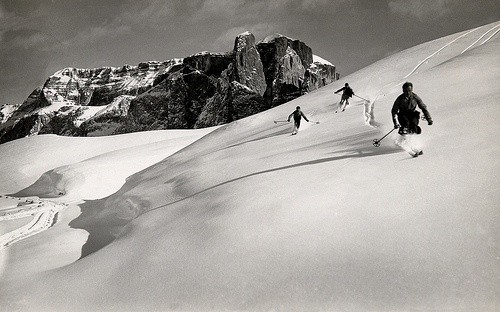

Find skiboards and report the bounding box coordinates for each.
[336,104,346,113]
[292,132,297,135]
[411,150,423,157]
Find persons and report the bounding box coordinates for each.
[391,82,433,159]
[335,83,355,113]
[287,105,309,135]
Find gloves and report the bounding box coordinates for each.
[428,122,433,125]
[288,120,290,122]
[394,125,398,129]
[307,120,309,122]
